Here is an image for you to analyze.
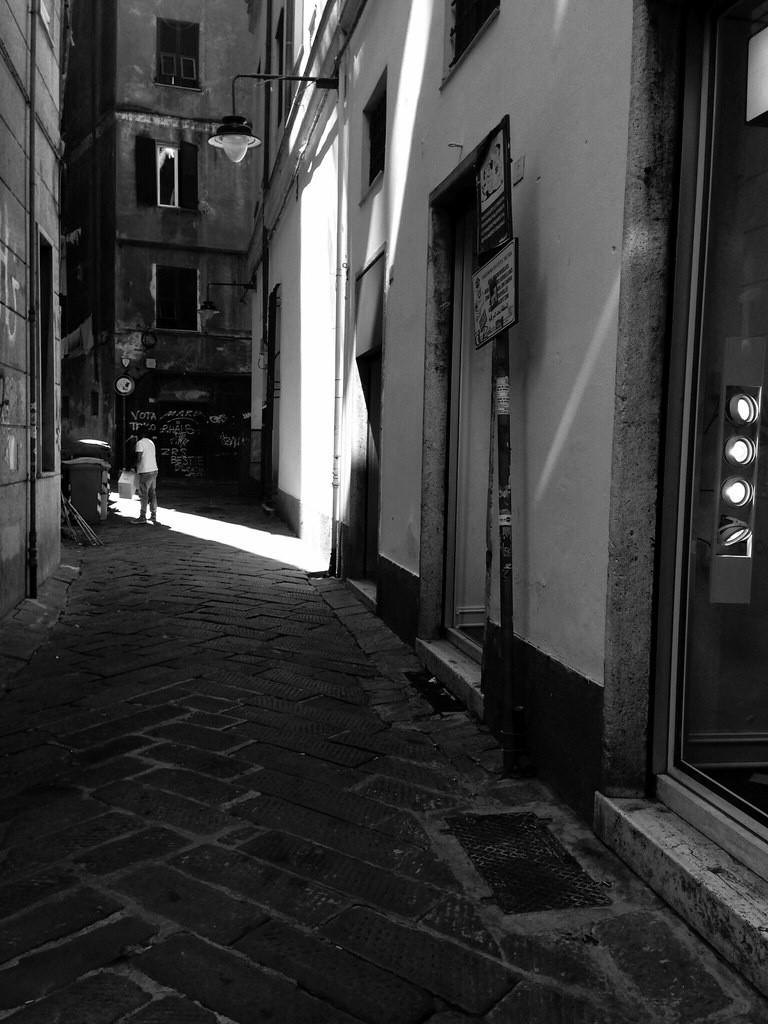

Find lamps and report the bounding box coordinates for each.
[197,274,257,320]
[208,74,339,163]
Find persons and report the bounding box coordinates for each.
[130,426,158,524]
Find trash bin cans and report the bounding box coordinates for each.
[74,439,110,464]
[61,457,111,524]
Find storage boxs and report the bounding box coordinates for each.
[118,468,140,499]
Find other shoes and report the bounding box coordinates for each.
[130,517,146,523]
[148,519,156,524]
